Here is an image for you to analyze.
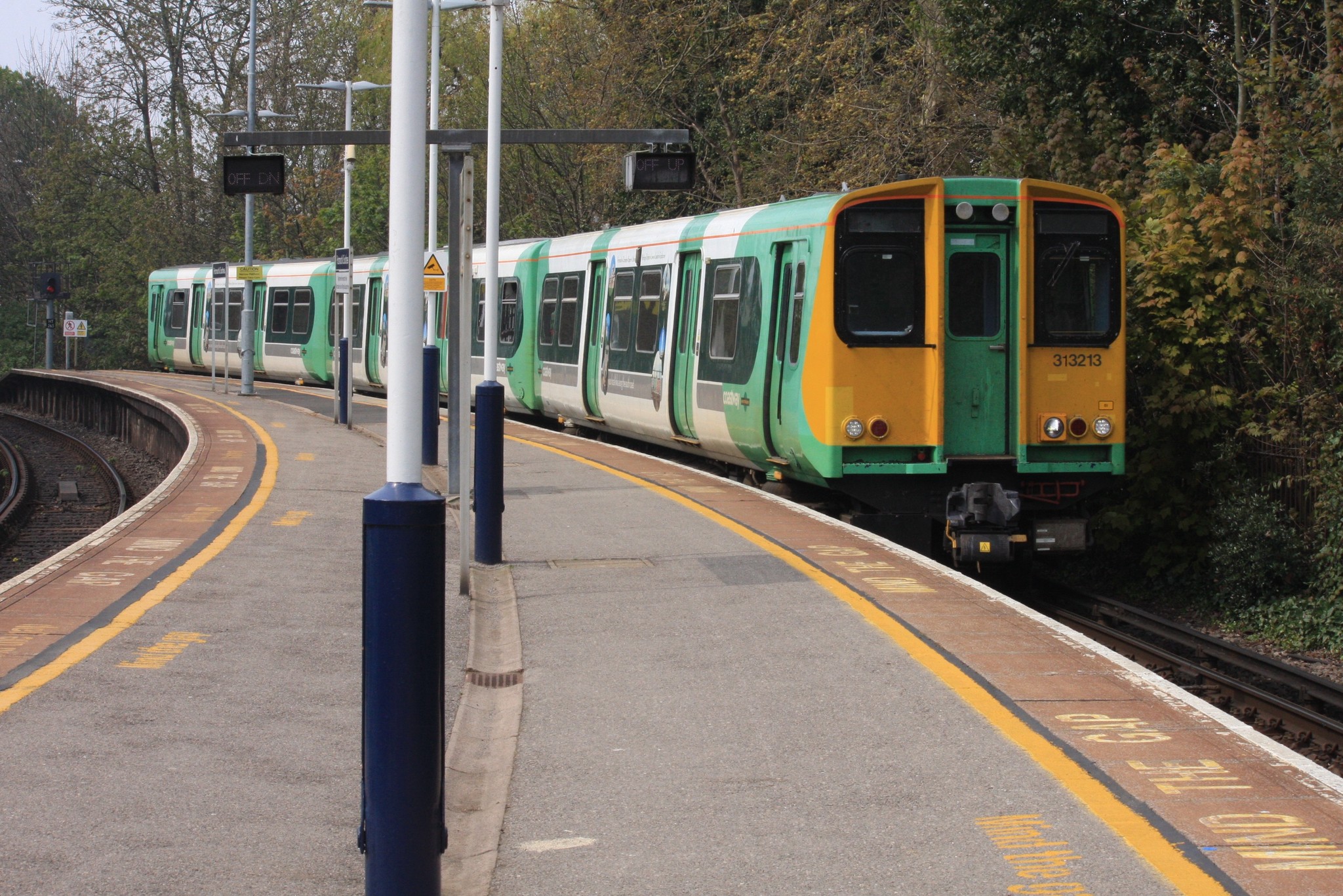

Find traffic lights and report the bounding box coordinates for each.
[42,272,61,296]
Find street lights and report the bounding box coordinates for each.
[203,109,303,396]
[295,79,393,425]
[358,0,492,467]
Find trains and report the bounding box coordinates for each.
[146,176,1129,583]
